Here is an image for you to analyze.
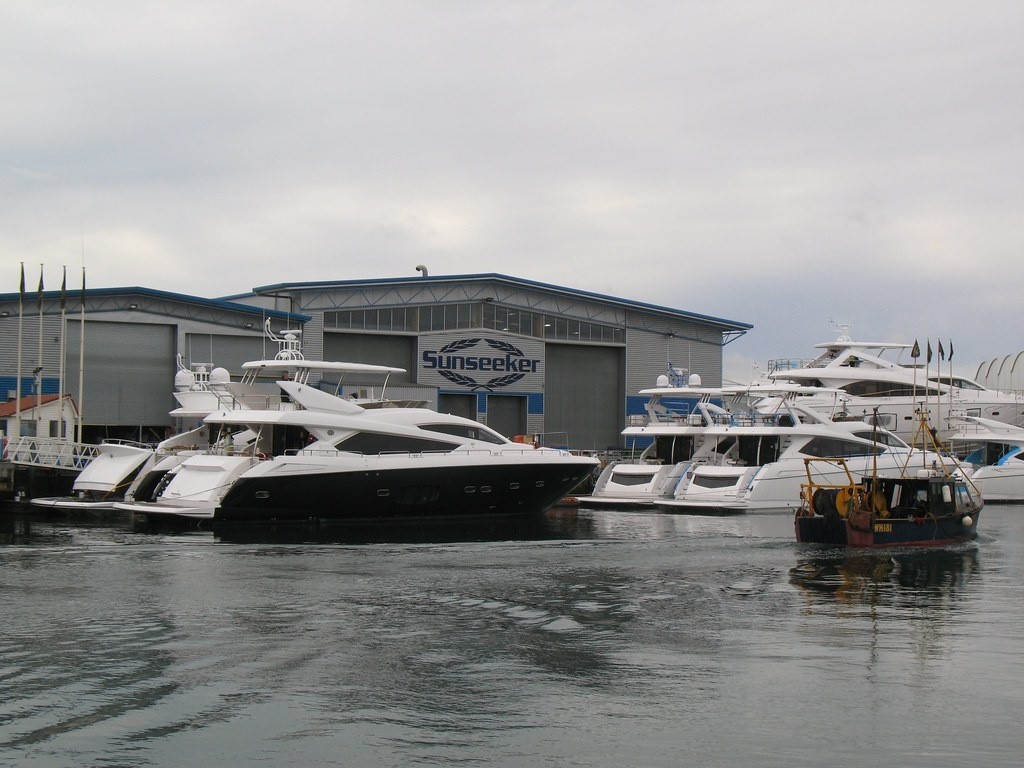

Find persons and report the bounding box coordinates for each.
[281,371,289,403]
[223,428,234,455]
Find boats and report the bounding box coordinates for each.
[792,400,985,549]
[564,324,1024,511]
[30,329,601,542]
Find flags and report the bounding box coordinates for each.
[911,340,920,357]
[948,342,953,361]
[61,275,66,308]
[928,342,932,362]
[38,274,44,309]
[20,264,25,310]
[939,341,944,360]
[80,273,86,306]
